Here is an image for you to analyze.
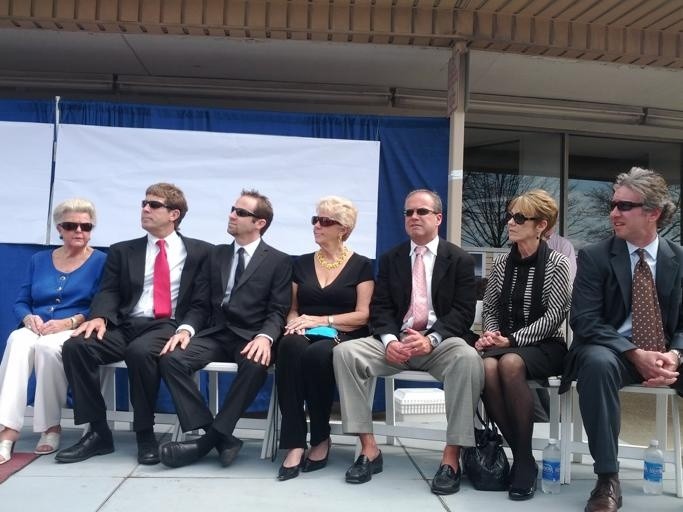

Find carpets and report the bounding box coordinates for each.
[0,453,40,484]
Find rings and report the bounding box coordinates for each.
[301,320,304,325]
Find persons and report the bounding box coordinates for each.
[475,191,569,500]
[158,189,293,468]
[567,166,683,512]
[498,190,577,316]
[0,197,107,468]
[50,182,214,464]
[330,189,482,497]
[276,198,376,479]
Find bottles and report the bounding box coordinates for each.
[541,439,561,495]
[642,439,665,495]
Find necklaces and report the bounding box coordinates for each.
[316,248,352,270]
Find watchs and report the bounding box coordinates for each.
[670,348,683,368]
[69,316,79,331]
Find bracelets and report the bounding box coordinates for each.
[328,314,336,328]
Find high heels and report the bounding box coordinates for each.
[278,448,305,480]
[302,436,332,472]
[34,424,61,455]
[0,440,16,464]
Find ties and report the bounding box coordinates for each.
[230,248,244,302]
[153,240,172,320]
[402,246,430,331]
[632,249,667,352]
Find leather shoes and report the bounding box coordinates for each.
[346,449,383,485]
[54,426,243,466]
[431,461,462,495]
[509,456,538,500]
[585,477,621,511]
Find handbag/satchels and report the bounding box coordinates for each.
[461,425,509,491]
[304,325,338,338]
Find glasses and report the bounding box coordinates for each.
[505,211,542,225]
[230,206,264,220]
[311,216,343,227]
[142,198,175,212]
[607,199,645,211]
[59,221,94,232]
[404,207,438,218]
